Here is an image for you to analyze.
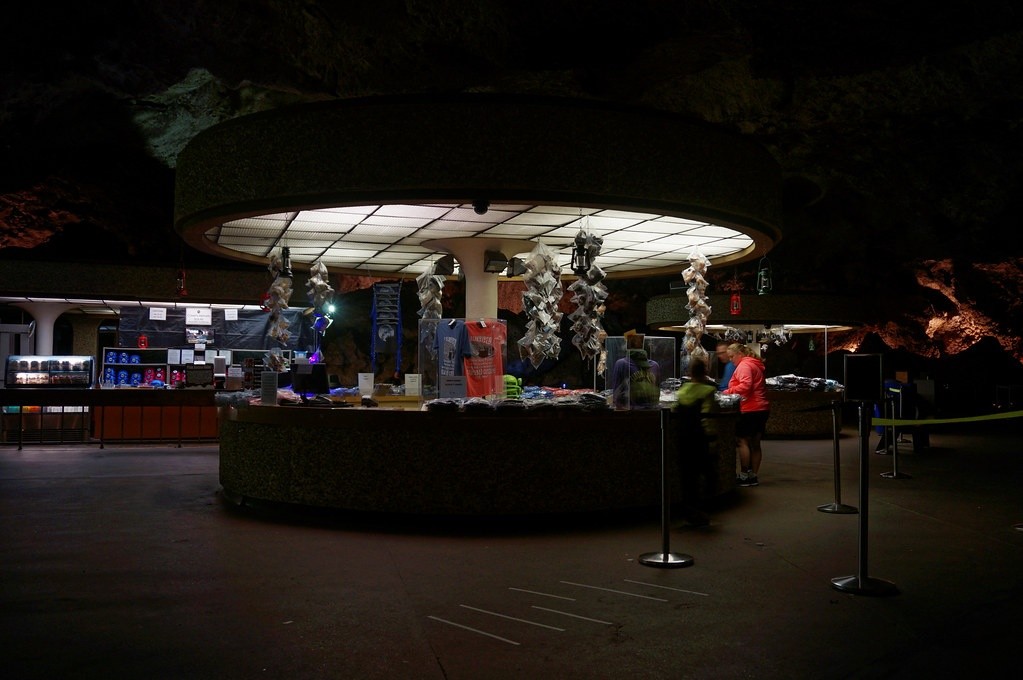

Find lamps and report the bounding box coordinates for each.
[431,254,455,276]
[484,250,528,278]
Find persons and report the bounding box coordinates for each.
[870,372,902,434]
[723,342,769,486]
[671,356,720,528]
[717,344,750,479]
[613,349,661,408]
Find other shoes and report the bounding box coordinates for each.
[736,475,760,486]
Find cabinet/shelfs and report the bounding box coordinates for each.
[4,355,93,388]
[102,347,170,386]
